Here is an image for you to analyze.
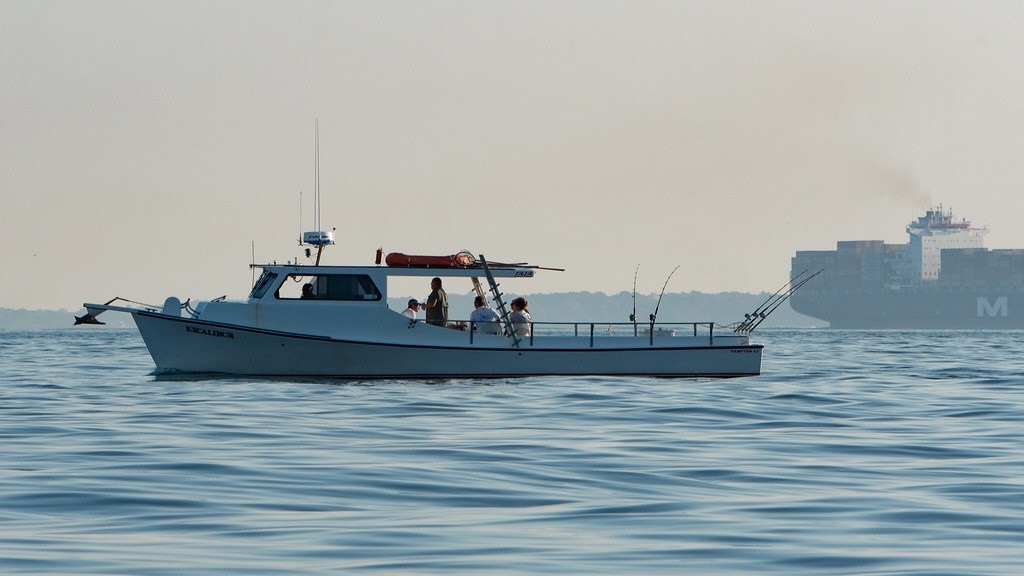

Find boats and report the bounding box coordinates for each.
[789,202,1024,328]
[73,116,825,378]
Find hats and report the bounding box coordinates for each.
[515,297,528,308]
[302,283,313,291]
[409,299,423,306]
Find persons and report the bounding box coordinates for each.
[299,282,316,299]
[470,296,501,335]
[401,299,420,320]
[504,297,532,336]
[421,277,449,327]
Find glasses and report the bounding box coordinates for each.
[511,303,515,305]
